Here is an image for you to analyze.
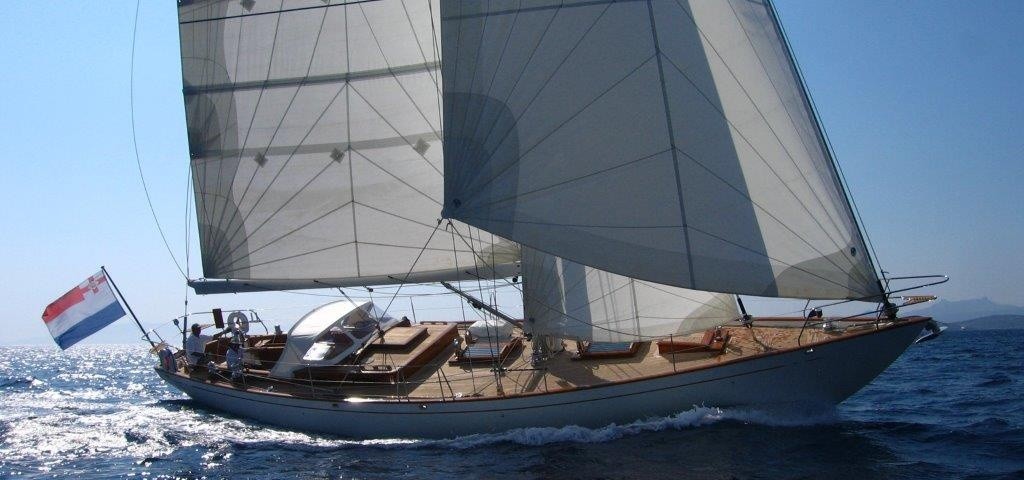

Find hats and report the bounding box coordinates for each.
[191,323,201,331]
[230,338,243,344]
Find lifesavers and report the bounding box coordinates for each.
[227,311,250,337]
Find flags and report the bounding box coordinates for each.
[41,270,127,350]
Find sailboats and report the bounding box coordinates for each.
[142,0,948,440]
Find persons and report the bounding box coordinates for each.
[226,336,244,368]
[186,323,232,370]
